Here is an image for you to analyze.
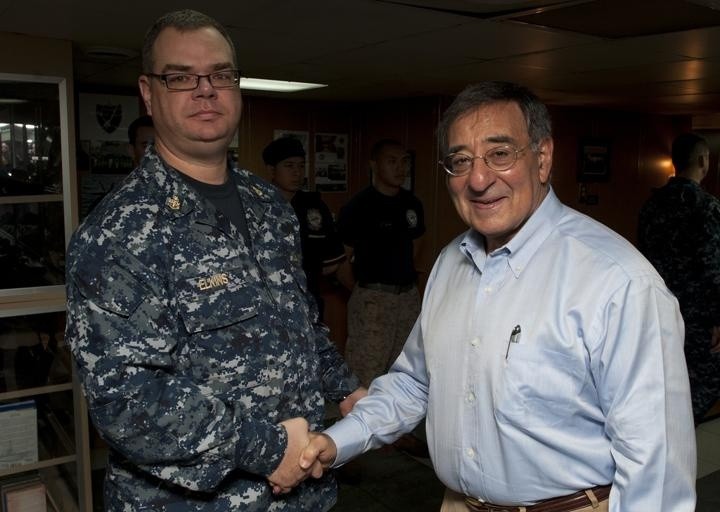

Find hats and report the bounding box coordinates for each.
[261,135,306,165]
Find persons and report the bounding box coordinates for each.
[126,115,157,167]
[634,131,719,424]
[257,135,336,330]
[65,10,370,509]
[336,142,428,459]
[272,80,696,512]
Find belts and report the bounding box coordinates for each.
[464,485,611,512]
[358,281,413,295]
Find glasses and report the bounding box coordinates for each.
[147,68,241,91]
[438,141,533,176]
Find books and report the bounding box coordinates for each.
[2,471,48,512]
